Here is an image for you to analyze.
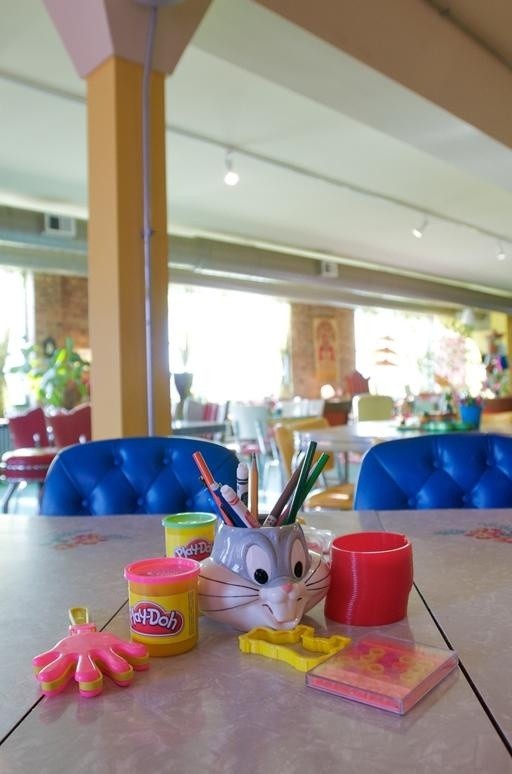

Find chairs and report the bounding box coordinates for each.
[38,437,241,516]
[274,418,354,512]
[352,433,512,510]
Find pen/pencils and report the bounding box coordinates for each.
[234,463,247,507]
[262,455,304,528]
[220,486,262,529]
[247,451,259,518]
[210,482,246,528]
[281,453,330,524]
[287,440,318,524]
[193,451,232,528]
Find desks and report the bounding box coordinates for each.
[0,506,511,774]
